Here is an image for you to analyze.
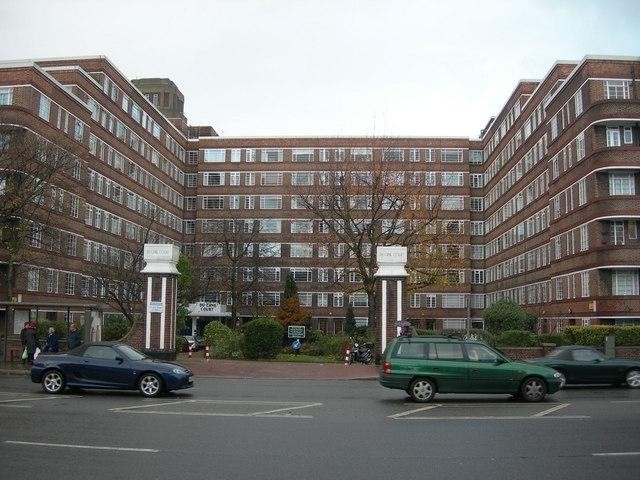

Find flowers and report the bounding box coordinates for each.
[394,320,411,328]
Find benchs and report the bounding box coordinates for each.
[9,347,25,360]
[35,340,70,364]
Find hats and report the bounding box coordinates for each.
[30,323,36,327]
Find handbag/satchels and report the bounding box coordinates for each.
[34,347,41,359]
[22,350,28,359]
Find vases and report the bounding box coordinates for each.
[401,326,409,333]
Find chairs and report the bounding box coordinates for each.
[469,350,479,360]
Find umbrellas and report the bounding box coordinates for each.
[394,319,412,328]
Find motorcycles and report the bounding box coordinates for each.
[349,337,374,365]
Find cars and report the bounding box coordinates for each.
[379,336,640,402]
[30,341,194,397]
[180,335,206,352]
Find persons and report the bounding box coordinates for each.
[20,322,29,364]
[67,324,81,350]
[24,322,41,364]
[42,327,59,353]
[398,326,412,338]
